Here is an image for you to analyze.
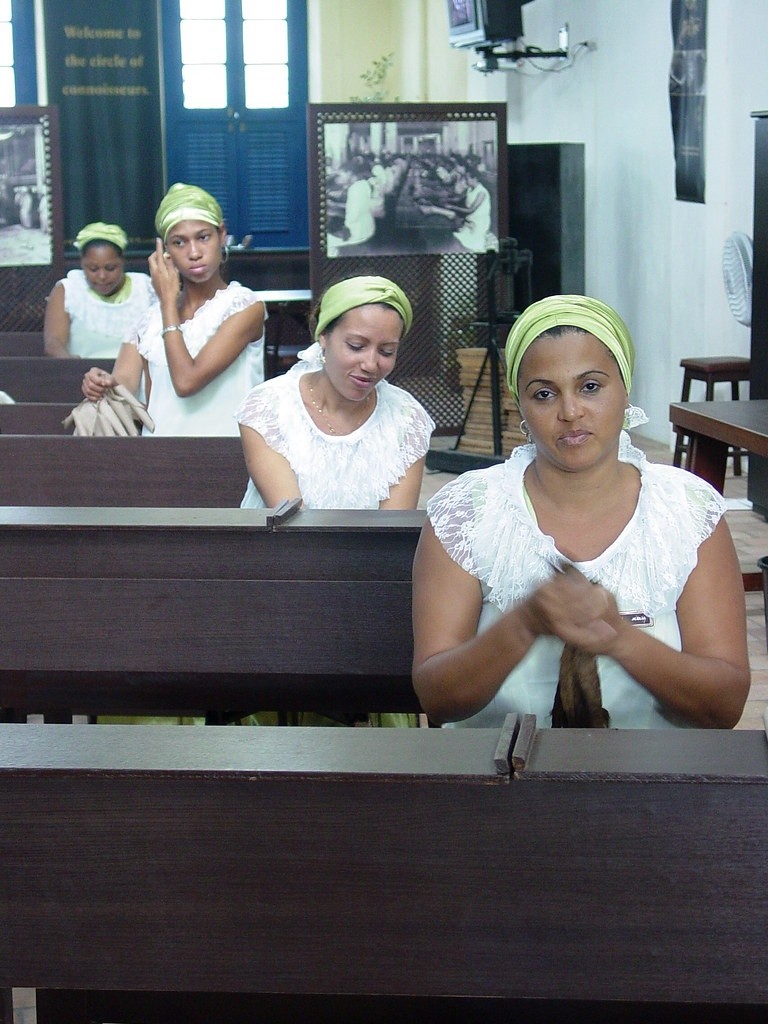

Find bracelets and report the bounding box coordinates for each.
[161,325,182,335]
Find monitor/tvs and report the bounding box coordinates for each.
[446,0,524,50]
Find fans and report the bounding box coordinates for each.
[722,231,753,327]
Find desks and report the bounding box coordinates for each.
[669,399,768,592]
[0,497,426,712]
[331,156,463,239]
[0,713,768,1024]
[0,331,249,508]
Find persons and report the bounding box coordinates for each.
[43,222,155,359]
[322,144,497,256]
[79,182,268,437]
[0,175,52,238]
[413,294,752,731]
[233,275,436,509]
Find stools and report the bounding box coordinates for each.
[485,232,500,254]
[672,356,752,476]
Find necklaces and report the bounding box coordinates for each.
[309,371,371,436]
